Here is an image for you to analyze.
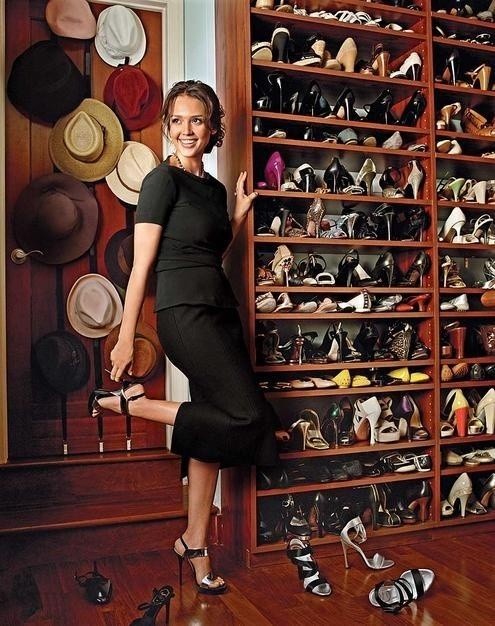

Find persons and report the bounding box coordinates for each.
[91,80,278,593]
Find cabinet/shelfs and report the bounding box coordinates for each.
[215,0,495,567]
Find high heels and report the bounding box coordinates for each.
[87,369,146,418]
[174,536,227,595]
[129,585,172,625]
[341,517,394,569]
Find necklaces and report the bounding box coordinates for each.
[170,149,205,178]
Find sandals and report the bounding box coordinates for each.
[75,564,112,605]
[369,568,434,613]
[285,539,332,596]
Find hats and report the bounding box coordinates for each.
[68,274,123,337]
[6,0,161,205]
[12,175,98,265]
[30,331,88,391]
[103,320,163,385]
[104,229,134,286]
